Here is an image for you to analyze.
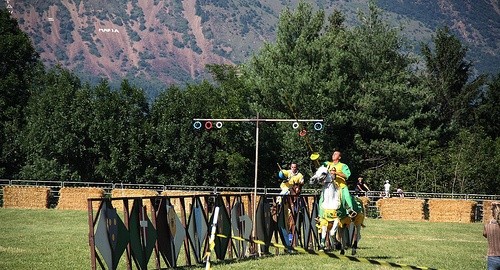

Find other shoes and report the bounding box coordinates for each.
[349,210,356,222]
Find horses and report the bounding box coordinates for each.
[310,165,367,255]
[271,180,304,250]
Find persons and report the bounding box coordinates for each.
[355,176,370,192]
[384,180,390,197]
[483,202,500,270]
[321,150,356,217]
[395,188,404,198]
[279,162,304,196]
